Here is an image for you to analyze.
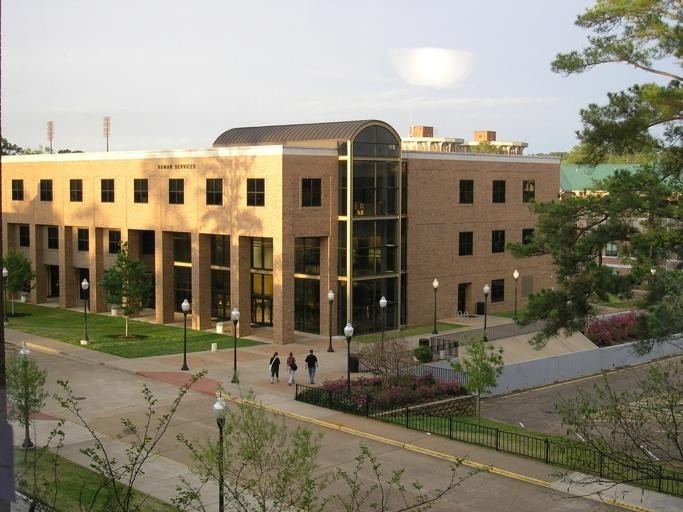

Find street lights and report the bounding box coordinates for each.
[181,298,241,383]
[2,267,8,322]
[431,277,439,334]
[212,390,226,512]
[80,278,89,340]
[483,282,490,342]
[512,269,519,316]
[18,340,33,447]
[327,289,387,395]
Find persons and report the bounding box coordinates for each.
[303,349,319,386]
[285,351,297,387]
[269,352,279,384]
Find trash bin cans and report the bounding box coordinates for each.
[20,295,27,302]
[211,343,218,352]
[111,306,118,317]
[477,302,484,315]
[216,323,223,333]
[418,338,429,346]
[348,354,358,371]
